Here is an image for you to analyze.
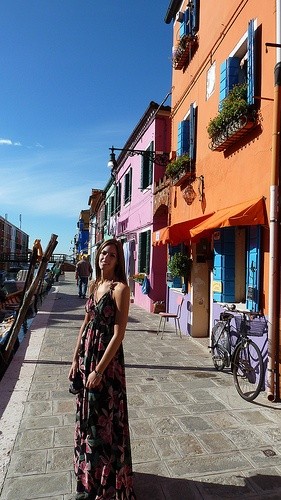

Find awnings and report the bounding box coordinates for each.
[152,212,215,246]
[189,196,265,237]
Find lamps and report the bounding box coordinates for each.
[183,174,206,206]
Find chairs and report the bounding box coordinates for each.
[157,295,184,340]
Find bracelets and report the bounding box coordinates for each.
[93,370,102,378]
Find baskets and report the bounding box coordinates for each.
[234,316,267,337]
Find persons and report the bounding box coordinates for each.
[76,256,93,299]
[68,240,135,500]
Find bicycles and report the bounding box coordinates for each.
[206,310,267,402]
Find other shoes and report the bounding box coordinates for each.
[81,295,85,299]
[79,295,81,297]
[71,491,89,500]
